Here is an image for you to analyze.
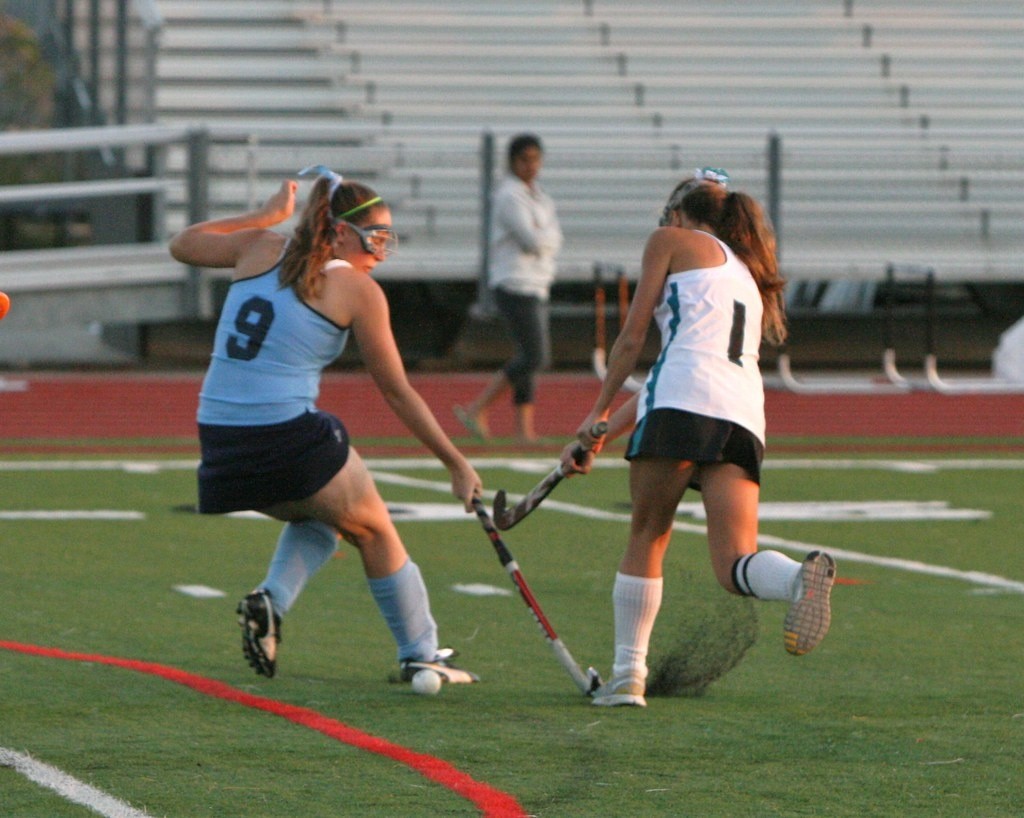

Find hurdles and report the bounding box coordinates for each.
[591,258,1024,399]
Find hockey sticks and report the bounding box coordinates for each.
[491,420,610,531]
[471,489,606,697]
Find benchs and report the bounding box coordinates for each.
[70,0,1024,317]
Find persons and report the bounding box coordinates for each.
[561,169,834,708]
[169,173,483,683]
[452,133,563,439]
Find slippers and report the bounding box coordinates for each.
[454,401,490,442]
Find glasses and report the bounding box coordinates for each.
[341,223,398,255]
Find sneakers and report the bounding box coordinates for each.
[784,553,836,656]
[399,645,480,684]
[237,590,281,678]
[592,674,647,706]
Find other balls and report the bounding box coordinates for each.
[411,668,442,696]
